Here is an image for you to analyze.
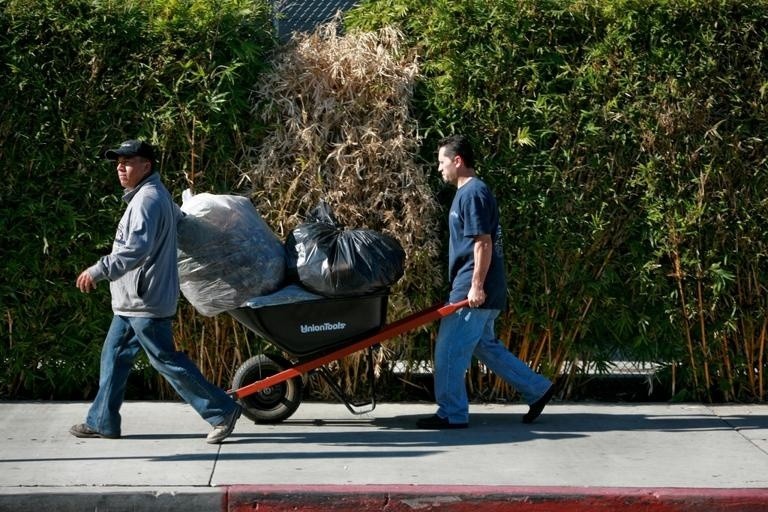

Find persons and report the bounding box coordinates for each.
[68,140,242,444]
[416,133,556,431]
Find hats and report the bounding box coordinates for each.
[104,139,158,162]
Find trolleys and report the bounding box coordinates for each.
[220,291,487,428]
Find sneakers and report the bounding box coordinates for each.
[417,411,469,428]
[68,421,122,440]
[521,381,560,423]
[205,404,242,443]
